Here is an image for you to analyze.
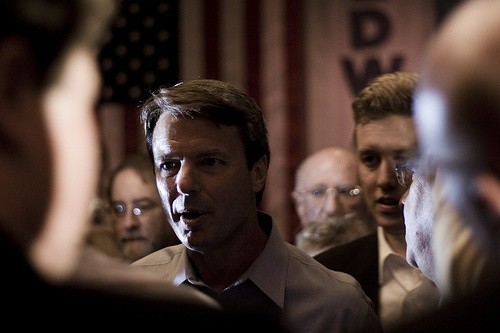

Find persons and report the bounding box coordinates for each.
[291,146,375,257]
[105,155,179,264]
[399,155,439,286]
[312,72,425,333]
[130,79,382,333]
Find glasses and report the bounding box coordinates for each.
[296,184,364,205]
[394,162,418,188]
